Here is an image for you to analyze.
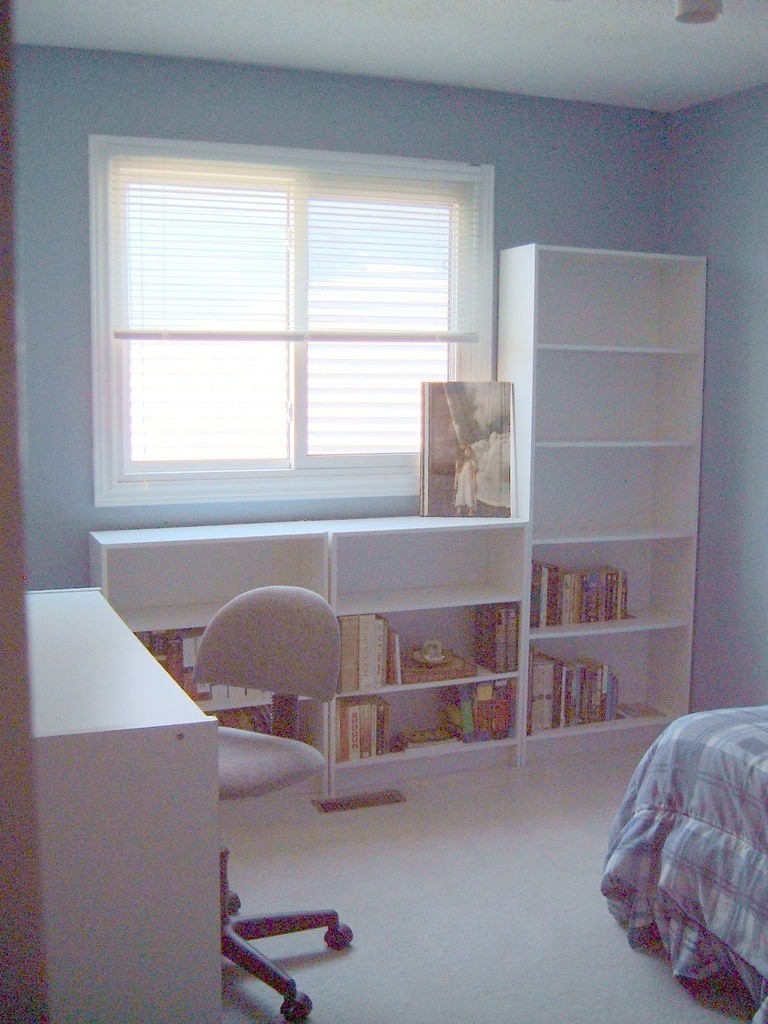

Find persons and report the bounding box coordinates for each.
[453,444,481,517]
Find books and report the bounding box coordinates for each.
[135,560,625,763]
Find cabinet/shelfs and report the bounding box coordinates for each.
[25,242,707,1024]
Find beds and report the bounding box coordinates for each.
[601,707,768,1024]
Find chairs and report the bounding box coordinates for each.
[191,585,354,1024]
